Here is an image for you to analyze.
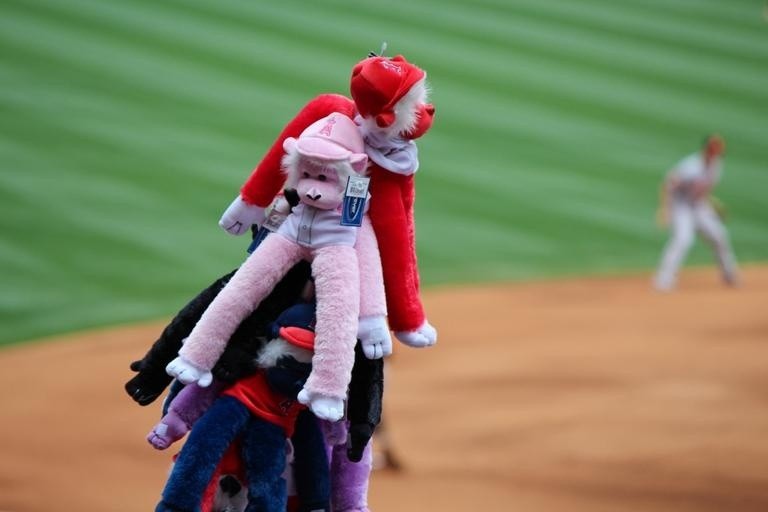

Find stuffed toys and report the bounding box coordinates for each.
[125,41,438,512]
[124,41,437,511]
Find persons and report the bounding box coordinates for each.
[651,134,742,293]
